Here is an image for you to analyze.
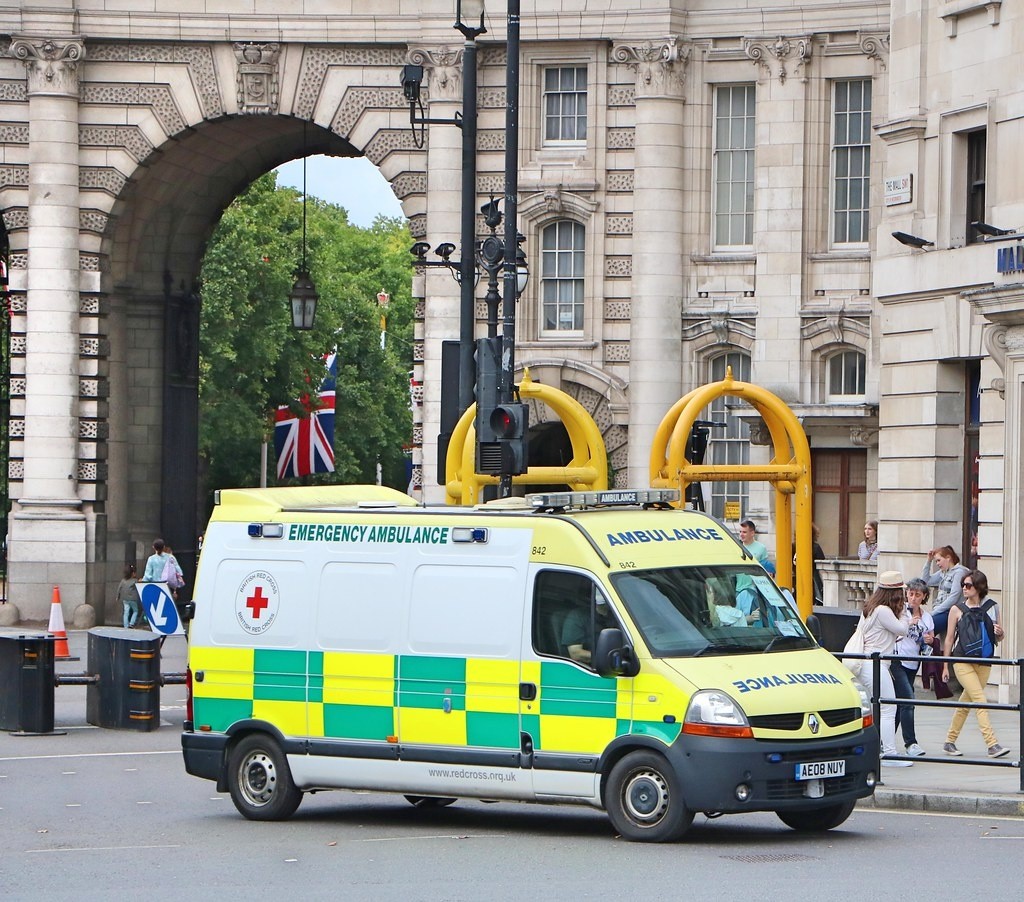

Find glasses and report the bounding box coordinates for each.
[961,582,973,590]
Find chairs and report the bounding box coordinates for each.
[549,609,571,657]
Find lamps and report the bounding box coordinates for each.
[286,120,322,331]
[969,220,1014,238]
[891,231,936,253]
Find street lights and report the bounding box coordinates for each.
[457,192,530,335]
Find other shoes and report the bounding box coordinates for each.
[881,757,913,767]
[128,625,133,628]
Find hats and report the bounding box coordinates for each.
[878,571,908,589]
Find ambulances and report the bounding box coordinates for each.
[180,484,881,842]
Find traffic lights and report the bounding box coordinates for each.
[489,403,529,439]
[471,336,502,444]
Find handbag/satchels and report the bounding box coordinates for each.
[161,555,179,590]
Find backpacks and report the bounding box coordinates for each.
[842,627,864,676]
[954,599,997,657]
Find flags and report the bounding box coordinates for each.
[274,343,337,480]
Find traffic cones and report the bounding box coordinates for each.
[47,585,80,661]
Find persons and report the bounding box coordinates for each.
[941,569,1010,758]
[734,561,776,623]
[115,565,139,628]
[792,522,825,603]
[859,571,914,766]
[141,538,183,600]
[735,520,767,596]
[856,519,877,559]
[889,578,934,756]
[921,548,969,634]
[562,587,618,664]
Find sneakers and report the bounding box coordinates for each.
[988,743,1010,758]
[943,742,963,756]
[906,744,925,756]
